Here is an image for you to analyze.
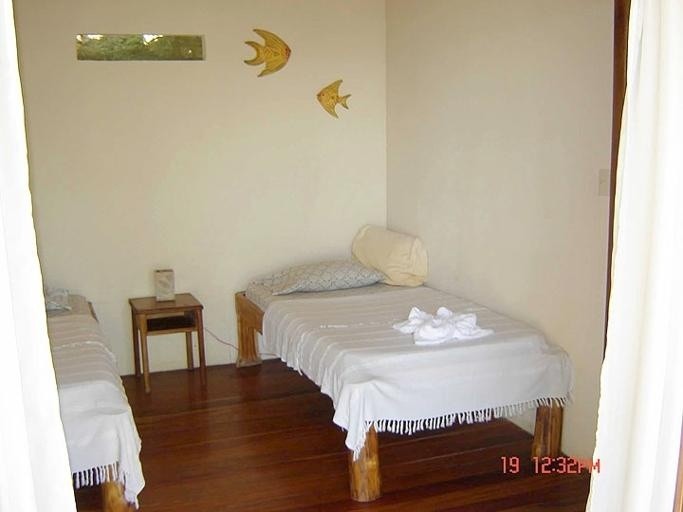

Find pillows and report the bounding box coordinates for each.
[43,289,72,311]
[350,227,429,288]
[252,256,380,293]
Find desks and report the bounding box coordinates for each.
[129,294,208,394]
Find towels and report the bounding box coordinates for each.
[393,306,492,347]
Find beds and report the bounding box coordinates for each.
[44,292,146,511]
[235,280,574,504]
